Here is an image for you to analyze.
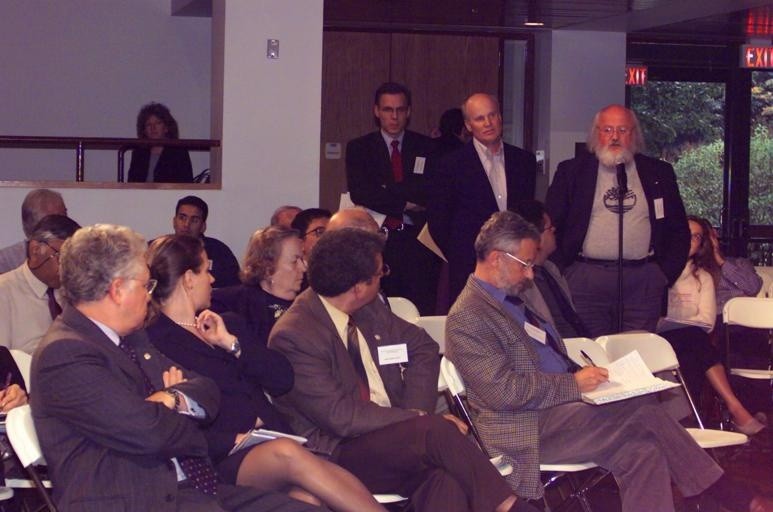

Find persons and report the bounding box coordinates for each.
[1,188,772,512]
[127,101,193,183]
[347,82,690,341]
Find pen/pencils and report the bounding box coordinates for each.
[580,349,612,384]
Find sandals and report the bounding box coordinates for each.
[729,412,765,435]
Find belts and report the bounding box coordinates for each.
[575,255,655,270]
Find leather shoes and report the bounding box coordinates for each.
[684,474,756,512]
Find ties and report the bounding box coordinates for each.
[504,296,562,357]
[484,151,506,215]
[383,140,405,232]
[119,339,219,497]
[347,315,371,401]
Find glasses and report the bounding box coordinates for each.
[300,227,327,239]
[596,125,637,134]
[488,247,535,270]
[120,277,159,296]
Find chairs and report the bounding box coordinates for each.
[7,406,56,512]
[596,333,749,450]
[564,337,607,367]
[440,357,611,512]
[388,298,419,325]
[722,296,773,429]
[755,266,773,296]
[372,464,512,512]
[10,349,33,394]
[411,315,462,420]
